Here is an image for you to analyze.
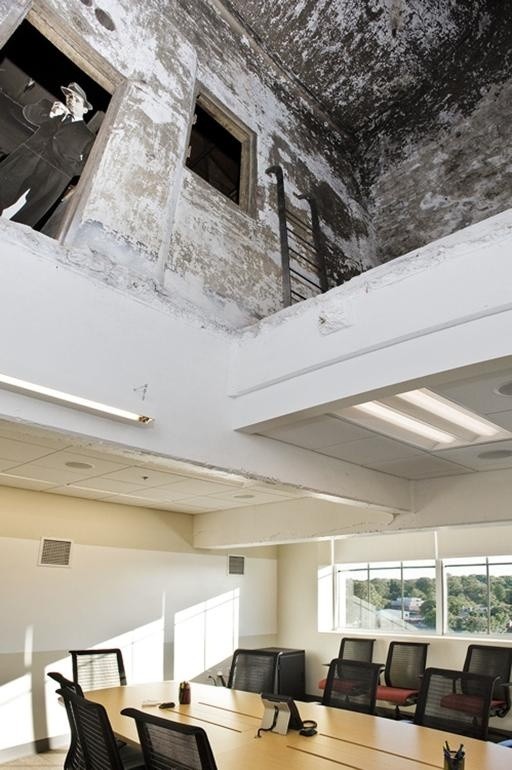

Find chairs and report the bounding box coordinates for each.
[439,640,510,736]
[119,706,220,768]
[319,657,387,716]
[317,638,379,707]
[410,666,496,742]
[225,647,284,696]
[54,688,135,768]
[66,647,127,690]
[374,640,430,720]
[48,670,88,770]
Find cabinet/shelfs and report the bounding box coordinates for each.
[254,644,309,702]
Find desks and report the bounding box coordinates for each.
[80,677,511,769]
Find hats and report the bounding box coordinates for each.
[60,82,93,110]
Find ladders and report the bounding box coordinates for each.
[265,165,328,307]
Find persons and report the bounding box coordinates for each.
[0,80,97,228]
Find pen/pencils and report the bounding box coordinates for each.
[443,741,465,770]
[180,681,189,704]
